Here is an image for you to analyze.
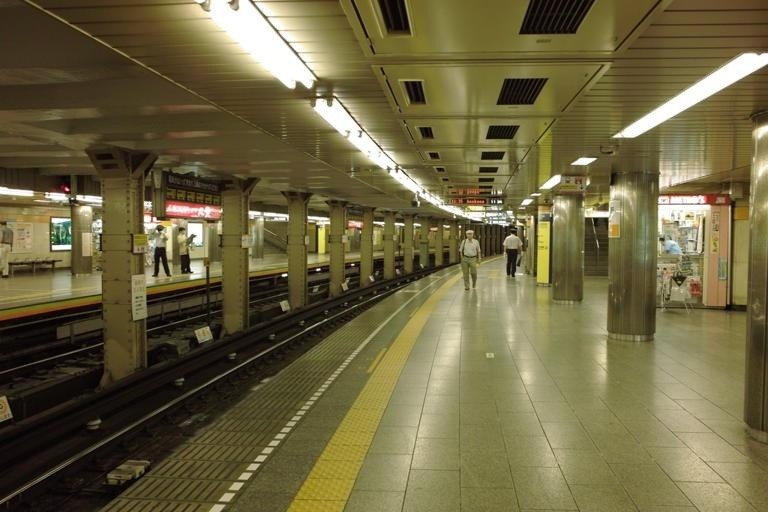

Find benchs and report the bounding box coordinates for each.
[8,259,63,276]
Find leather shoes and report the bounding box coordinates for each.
[182,271,193,274]
[507,272,515,277]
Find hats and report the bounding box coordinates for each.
[466,230,474,234]
[510,229,517,234]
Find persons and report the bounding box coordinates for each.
[660,238,683,271]
[458,229,482,290]
[0,219,13,277]
[150,225,174,277]
[503,226,524,277]
[176,227,194,274]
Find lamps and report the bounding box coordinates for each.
[0,183,357,223]
[200,0,495,221]
[495,50,767,223]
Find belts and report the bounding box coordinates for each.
[464,255,477,258]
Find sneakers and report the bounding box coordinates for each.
[152,274,172,277]
[465,282,477,290]
